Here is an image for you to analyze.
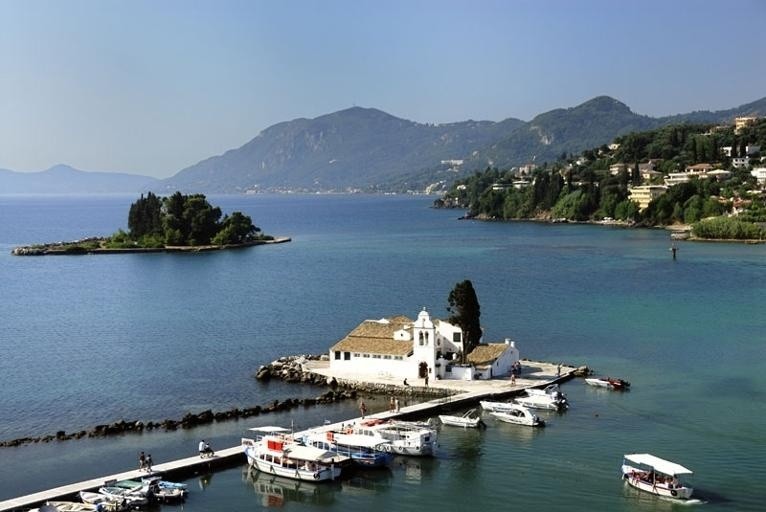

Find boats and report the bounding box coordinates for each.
[396,454,431,489]
[515,393,568,413]
[353,418,441,457]
[584,376,631,391]
[622,450,694,500]
[525,383,560,396]
[344,462,394,497]
[245,469,343,508]
[479,400,542,429]
[295,432,396,466]
[23,478,190,512]
[244,438,349,481]
[440,408,482,428]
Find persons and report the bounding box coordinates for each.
[395,397,400,413]
[390,397,395,413]
[359,400,365,419]
[139,452,146,471]
[199,440,206,458]
[404,378,409,386]
[145,455,153,472]
[511,361,521,384]
[205,443,214,454]
[424,369,429,387]
